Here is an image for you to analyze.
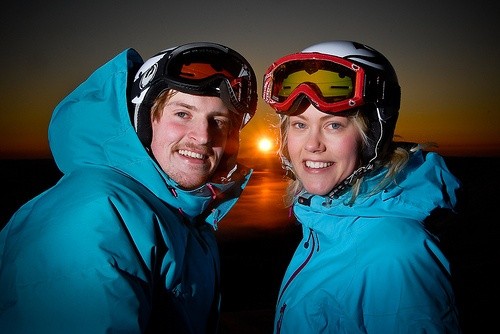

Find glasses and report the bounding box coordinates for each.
[130,42,259,130]
[262,51,389,115]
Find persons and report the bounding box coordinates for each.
[262,41,463,334]
[0,42,257,334]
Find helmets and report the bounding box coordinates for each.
[301,41,401,162]
[131,46,179,148]
